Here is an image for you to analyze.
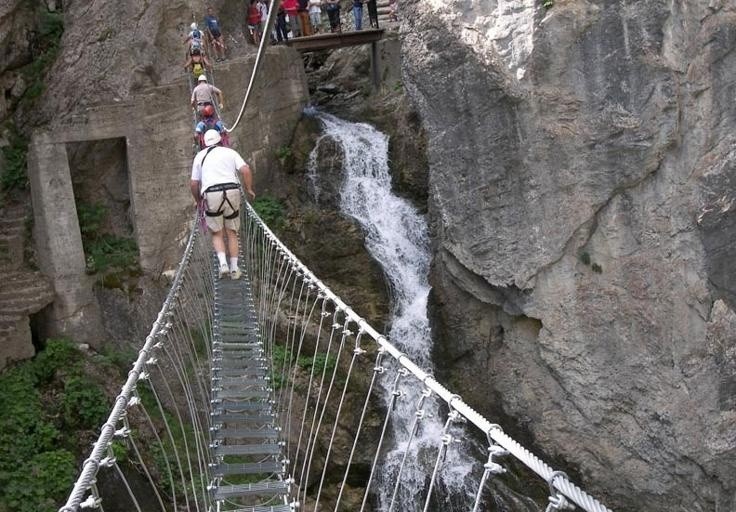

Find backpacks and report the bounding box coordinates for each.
[193,62,204,78]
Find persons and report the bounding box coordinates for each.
[204,6,226,62]
[191,104,230,153]
[271,0,289,45]
[321,0,341,33]
[255,0,268,41]
[296,0,310,37]
[246,0,261,46]
[364,0,379,29]
[349,0,365,31]
[181,36,208,62]
[189,73,224,120]
[182,47,212,81]
[182,21,207,46]
[188,128,257,282]
[307,0,324,35]
[389,0,396,15]
[279,0,304,37]
[387,0,399,23]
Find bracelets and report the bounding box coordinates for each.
[257,11,260,13]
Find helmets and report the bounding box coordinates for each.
[190,23,202,55]
[203,129,221,147]
[203,105,215,116]
[198,75,207,82]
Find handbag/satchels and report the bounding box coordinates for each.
[326,3,342,10]
[247,24,256,30]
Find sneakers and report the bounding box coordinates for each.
[268,32,313,46]
[218,264,229,279]
[230,267,242,280]
[215,57,226,62]
